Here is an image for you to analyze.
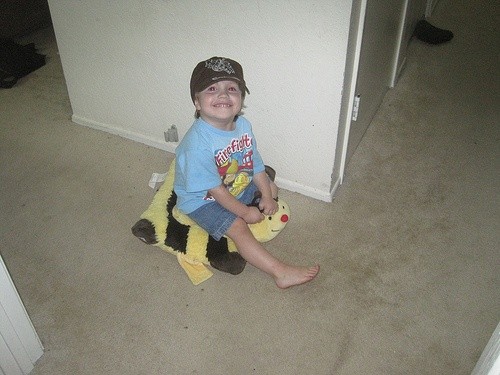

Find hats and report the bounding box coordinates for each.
[190,57,250,104]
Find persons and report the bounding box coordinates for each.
[172,56,321,289]
